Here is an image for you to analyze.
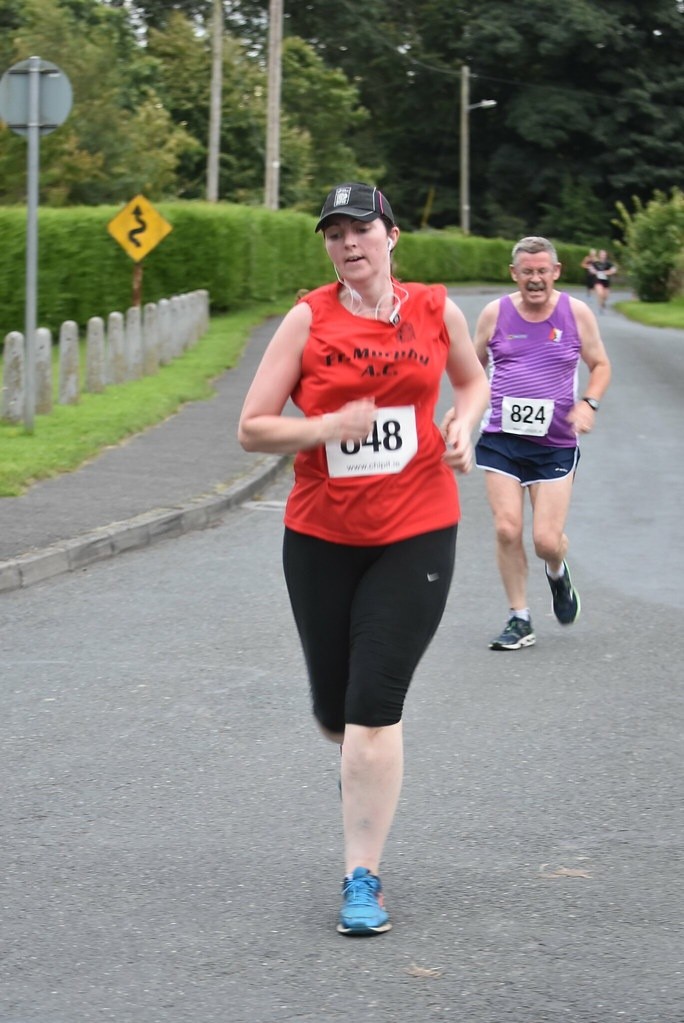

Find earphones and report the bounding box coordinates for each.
[388,236,393,251]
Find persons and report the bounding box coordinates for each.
[581,248,616,316]
[236,183,493,938]
[440,236,612,651]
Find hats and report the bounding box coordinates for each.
[315,181,394,232]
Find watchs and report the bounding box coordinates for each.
[580,396,601,413]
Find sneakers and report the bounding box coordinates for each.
[335,868,391,935]
[544,559,580,626]
[489,613,537,651]
